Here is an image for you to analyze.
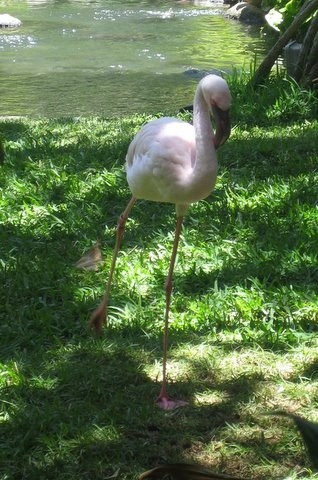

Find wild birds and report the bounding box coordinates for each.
[88,74,232,412]
